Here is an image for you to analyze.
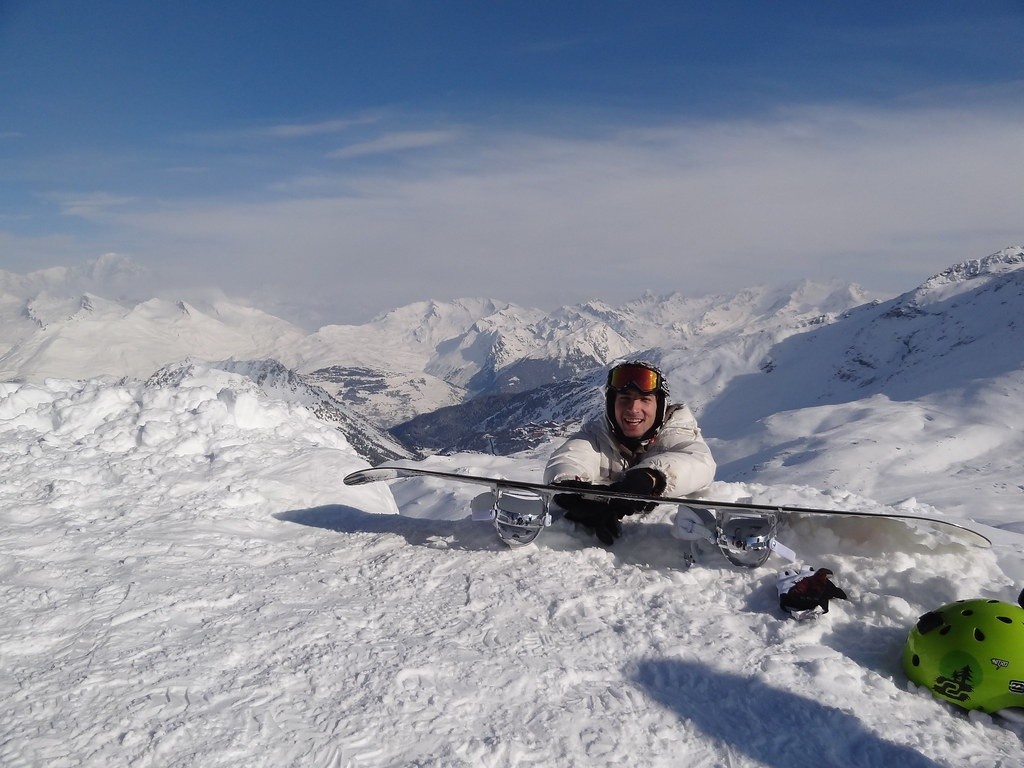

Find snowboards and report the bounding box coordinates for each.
[343,466,994,571]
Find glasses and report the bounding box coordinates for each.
[609,366,659,394]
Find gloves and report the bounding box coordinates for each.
[608,469,654,519]
[556,492,622,546]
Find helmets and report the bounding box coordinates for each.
[898,599,1023,712]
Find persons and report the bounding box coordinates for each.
[543,359,717,545]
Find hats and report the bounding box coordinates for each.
[607,360,670,449]
